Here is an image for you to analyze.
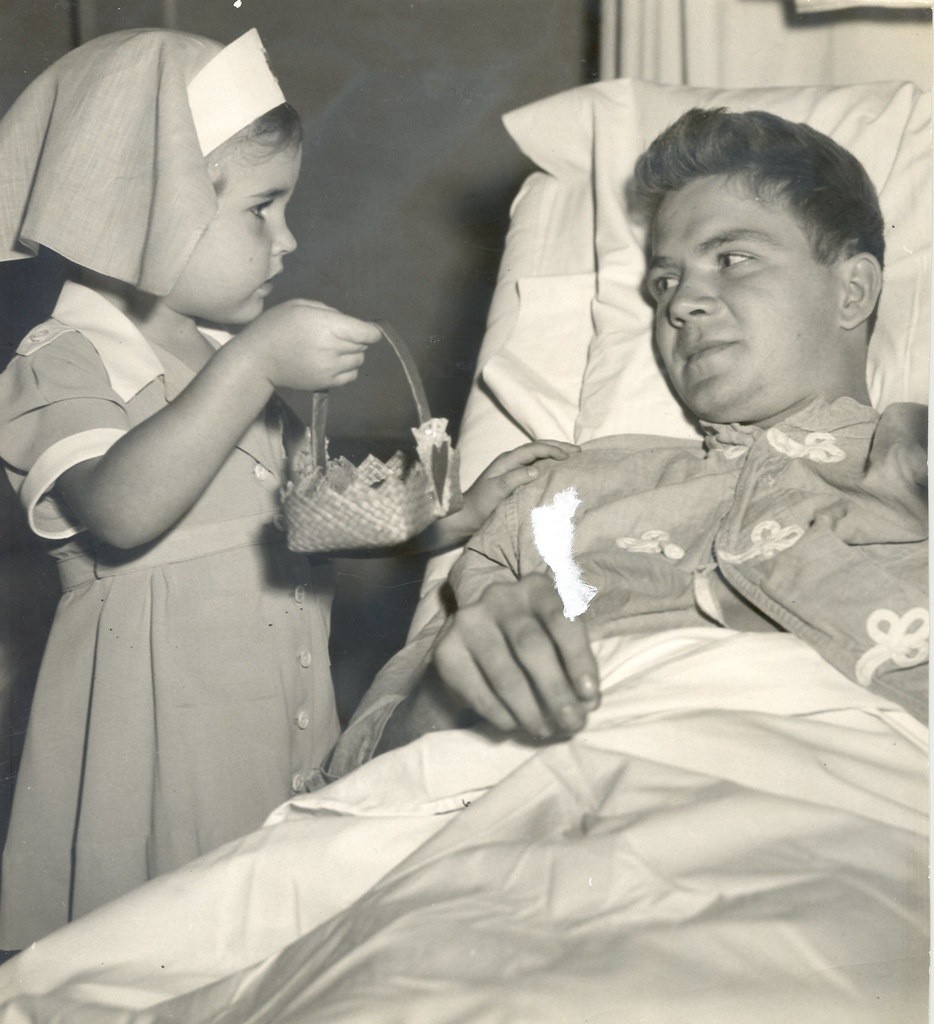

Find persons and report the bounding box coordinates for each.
[0,27,583,960]
[322,108,928,784]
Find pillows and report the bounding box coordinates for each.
[499,80,934,456]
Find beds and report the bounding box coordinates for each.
[0,71,933,1024]
